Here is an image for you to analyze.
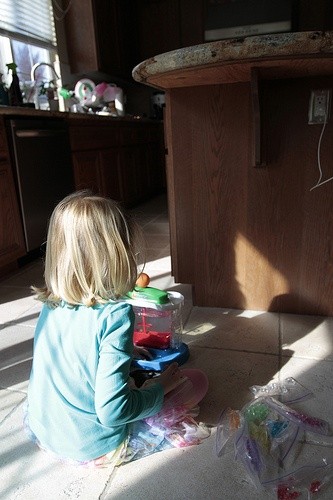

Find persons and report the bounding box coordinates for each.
[22,189,208,467]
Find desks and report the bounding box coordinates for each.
[131,29,333,316]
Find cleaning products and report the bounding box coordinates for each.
[47,81,54,99]
[60,84,69,99]
[39,81,48,95]
[6,63,23,106]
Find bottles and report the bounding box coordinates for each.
[38,88,50,111]
[47,81,59,111]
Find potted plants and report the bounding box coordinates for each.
[39,78,59,111]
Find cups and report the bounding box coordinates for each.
[19,80,35,103]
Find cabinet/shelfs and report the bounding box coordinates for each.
[0,104,166,277]
[64,0,210,91]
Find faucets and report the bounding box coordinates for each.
[31,63,59,109]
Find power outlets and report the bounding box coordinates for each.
[307,88,330,125]
